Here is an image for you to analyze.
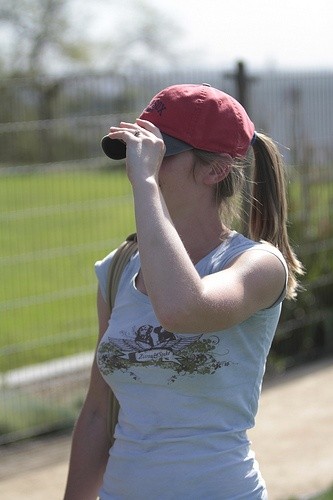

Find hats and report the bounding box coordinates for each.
[100,82,256,161]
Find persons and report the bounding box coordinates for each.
[63,83,308,500]
